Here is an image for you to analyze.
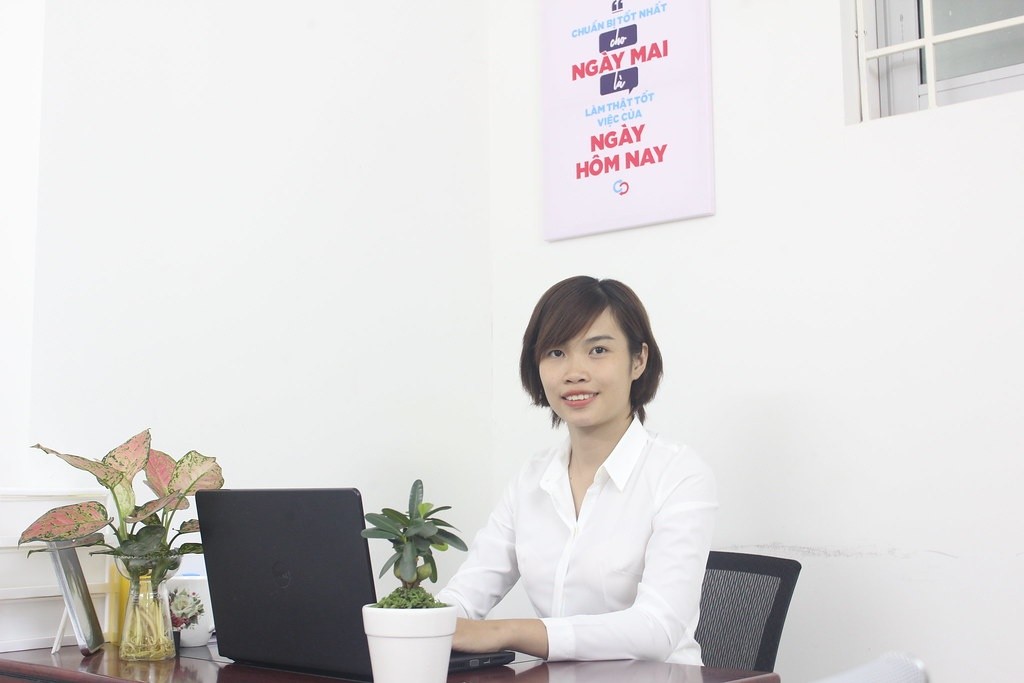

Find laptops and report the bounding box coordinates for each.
[195,489,515,677]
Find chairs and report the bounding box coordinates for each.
[695,551,802,672]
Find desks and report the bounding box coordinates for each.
[0,635,782,683]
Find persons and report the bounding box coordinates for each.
[432,276,709,667]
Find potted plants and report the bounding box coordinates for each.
[358,478,469,683]
[17,428,225,662]
